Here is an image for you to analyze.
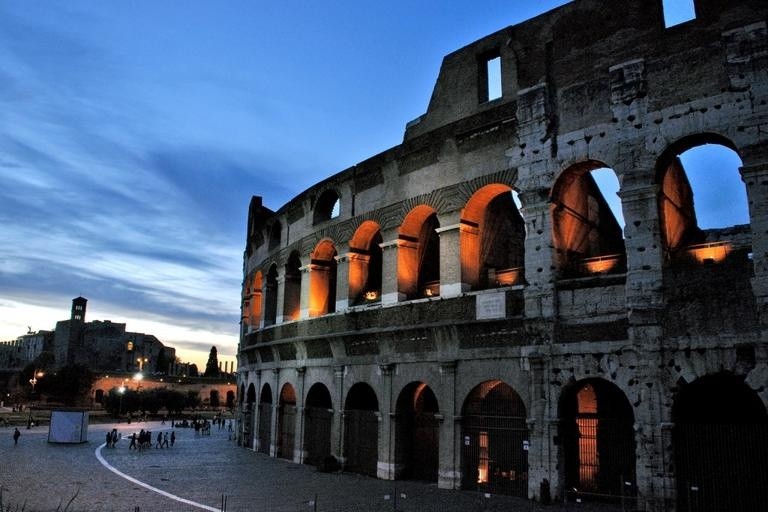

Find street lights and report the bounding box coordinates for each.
[136,358,147,387]
[118,381,124,423]
[28,368,43,428]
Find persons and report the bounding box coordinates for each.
[5,418,9,427]
[12,402,22,412]
[13,428,19,445]
[27,416,31,429]
[24,414,27,425]
[31,417,33,427]
[105,412,233,450]
[0,417,4,427]
